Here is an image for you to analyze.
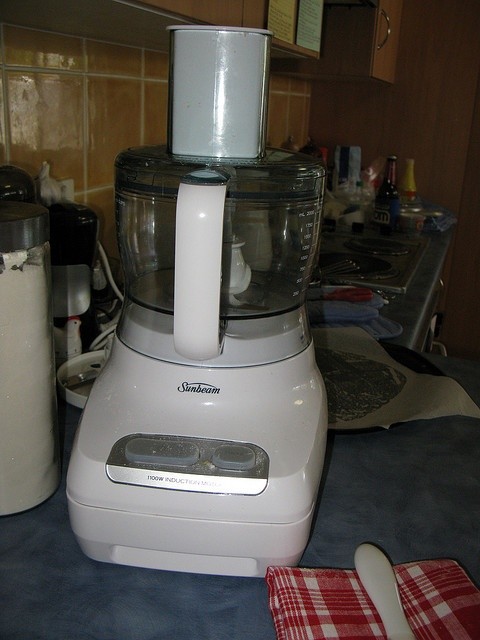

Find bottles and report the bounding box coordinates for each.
[372,155,400,236]
[402,159,417,206]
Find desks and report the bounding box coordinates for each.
[304,192,456,357]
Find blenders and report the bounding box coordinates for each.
[65,24,329,579]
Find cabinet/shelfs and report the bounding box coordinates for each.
[271,0,403,85]
[1,0,322,61]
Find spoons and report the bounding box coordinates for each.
[354,543,414,639]
